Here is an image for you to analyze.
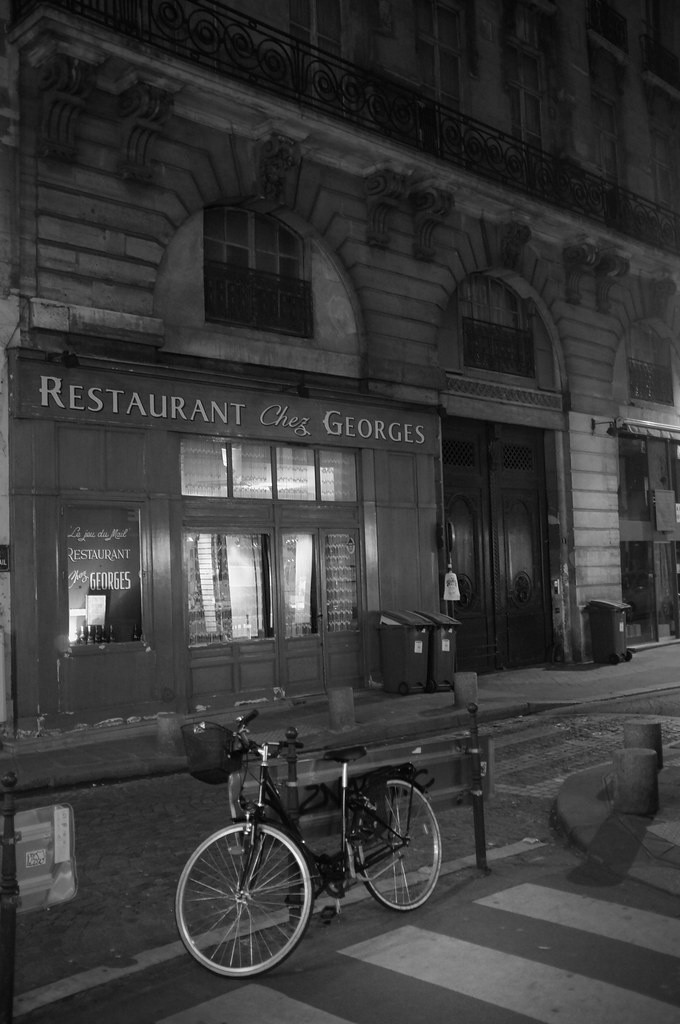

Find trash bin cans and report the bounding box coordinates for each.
[376,608,436,696]
[581,599,633,666]
[413,610,463,694]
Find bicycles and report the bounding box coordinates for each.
[173,708,442,979]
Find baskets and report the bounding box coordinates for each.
[180,722,243,786]
